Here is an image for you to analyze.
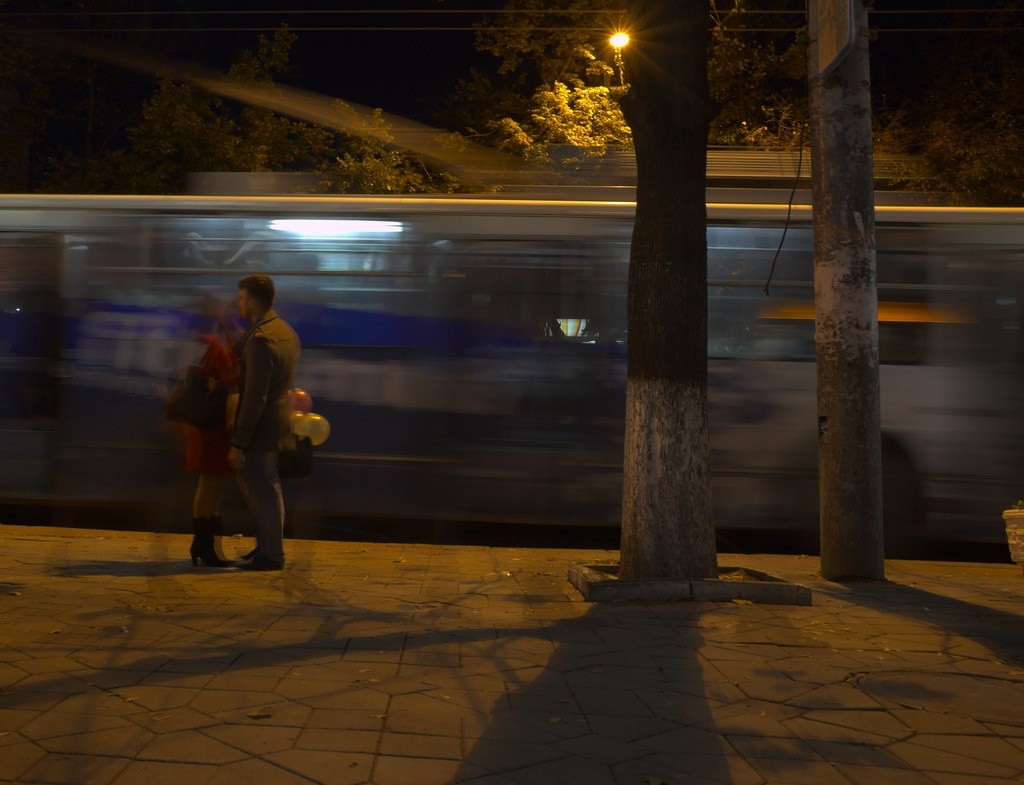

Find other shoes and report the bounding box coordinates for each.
[236,550,286,570]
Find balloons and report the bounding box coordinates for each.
[279,388,330,448]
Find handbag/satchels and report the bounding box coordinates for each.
[165,364,227,431]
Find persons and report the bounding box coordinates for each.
[180,273,301,571]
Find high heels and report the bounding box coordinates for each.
[191,515,231,567]
